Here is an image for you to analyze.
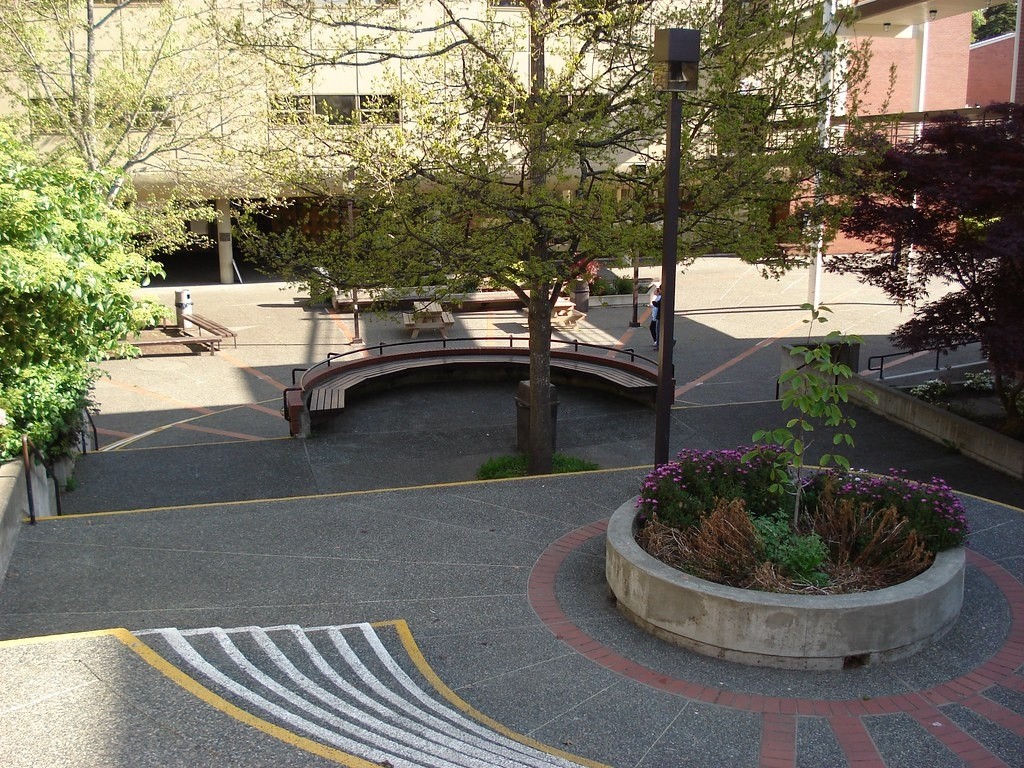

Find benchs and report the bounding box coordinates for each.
[566,309,587,333]
[116,336,223,357]
[441,311,456,337]
[522,307,529,314]
[310,354,658,426]
[783,253,826,265]
[403,313,416,340]
[180,313,238,352]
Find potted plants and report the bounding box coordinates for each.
[604,300,969,672]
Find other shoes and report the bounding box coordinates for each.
[653,348,658,351]
[652,342,657,347]
[673,340,676,347]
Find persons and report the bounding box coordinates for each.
[649,285,676,351]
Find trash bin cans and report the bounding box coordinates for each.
[569,278,590,313]
[174,288,194,328]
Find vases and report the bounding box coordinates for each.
[569,279,590,313]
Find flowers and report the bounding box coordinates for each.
[566,254,603,284]
[630,442,975,550]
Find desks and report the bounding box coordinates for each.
[410,301,450,338]
[550,295,579,332]
[775,244,834,265]
